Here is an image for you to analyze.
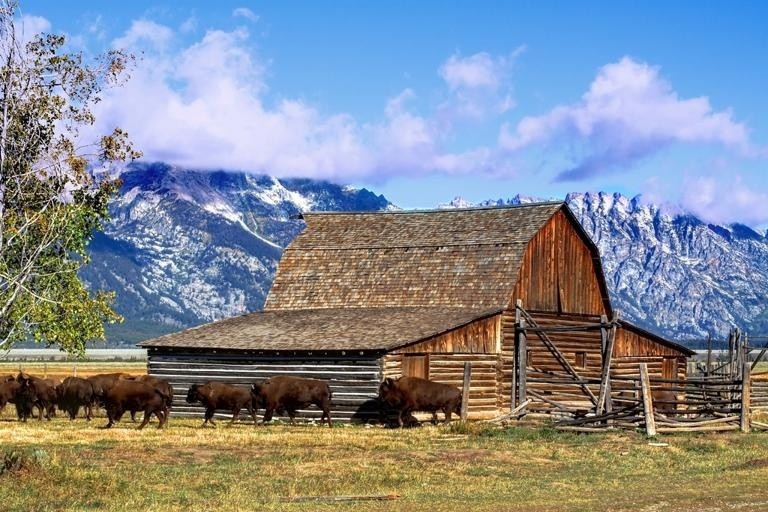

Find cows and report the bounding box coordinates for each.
[379,375,463,429]
[250,374,333,428]
[186,380,258,429]
[0,368,175,430]
